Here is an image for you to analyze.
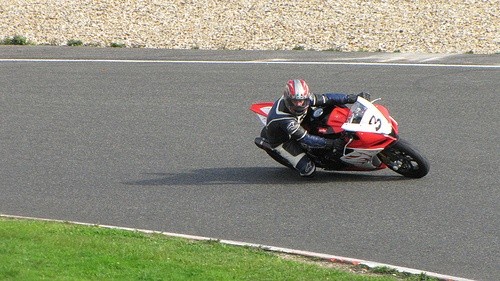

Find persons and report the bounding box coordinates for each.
[254,79,370,178]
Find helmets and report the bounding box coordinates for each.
[284,79,310,116]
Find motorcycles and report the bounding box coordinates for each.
[250,96,430,180]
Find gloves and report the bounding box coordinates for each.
[325,139,344,152]
[348,92,371,104]
